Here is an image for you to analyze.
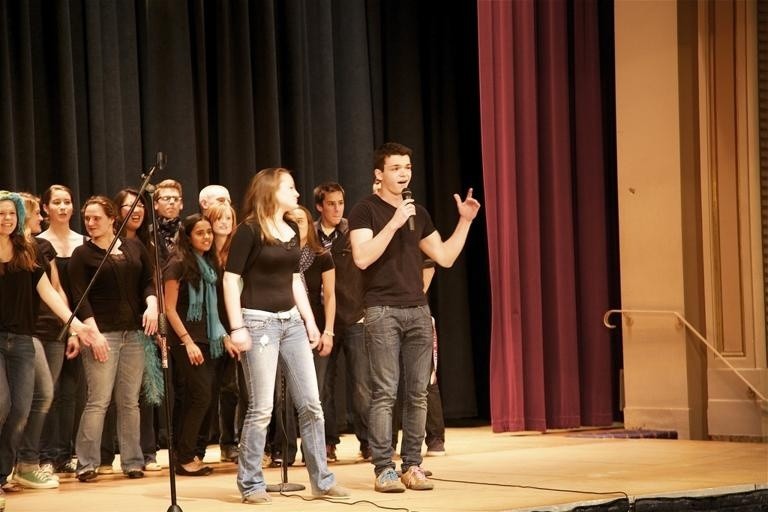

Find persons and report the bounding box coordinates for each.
[0,141,482,512]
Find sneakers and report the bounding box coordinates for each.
[42,463,57,482]
[145,463,162,471]
[54,462,78,478]
[326,485,351,498]
[244,490,271,505]
[374,468,406,493]
[326,451,337,462]
[402,465,433,490]
[13,468,59,488]
[426,443,445,457]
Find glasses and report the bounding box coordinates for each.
[156,195,182,201]
[120,202,144,207]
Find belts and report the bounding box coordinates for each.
[241,305,298,319]
[389,305,419,309]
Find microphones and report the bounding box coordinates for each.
[400,187,414,231]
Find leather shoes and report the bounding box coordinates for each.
[79,471,96,481]
[128,470,143,478]
[175,464,212,476]
